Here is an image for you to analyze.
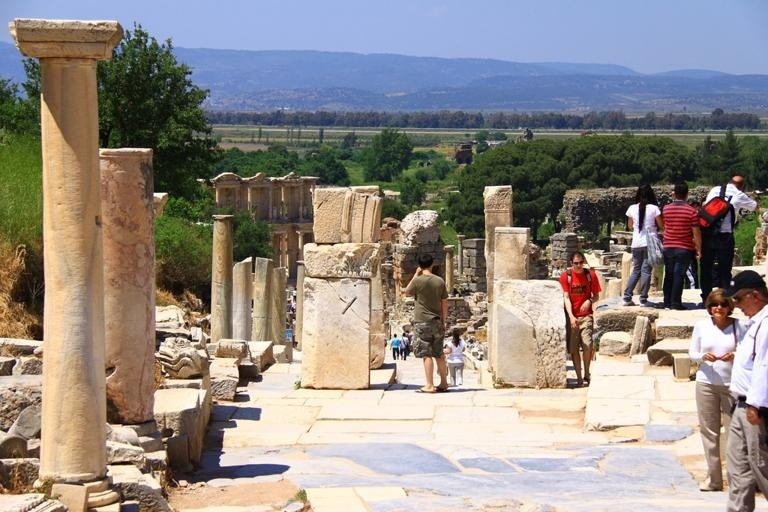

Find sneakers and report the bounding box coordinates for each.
[699,476,715,491]
[623,300,640,306]
[664,304,689,312]
[577,377,583,387]
[583,377,590,386]
[640,302,655,307]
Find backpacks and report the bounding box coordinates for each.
[696,186,735,236]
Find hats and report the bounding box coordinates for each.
[722,270,765,298]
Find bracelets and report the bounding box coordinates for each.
[586,297,594,305]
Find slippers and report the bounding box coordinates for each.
[414,386,437,392]
[436,387,450,392]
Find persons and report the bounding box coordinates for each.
[621,184,664,307]
[285,288,298,348]
[661,181,702,310]
[682,199,699,290]
[559,251,601,388]
[399,333,409,360]
[720,269,768,512]
[697,175,760,308]
[687,288,745,491]
[389,334,400,360]
[403,254,449,393]
[444,328,466,387]
[404,331,411,355]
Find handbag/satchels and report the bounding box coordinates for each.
[646,225,666,268]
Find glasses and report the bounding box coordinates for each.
[574,261,584,265]
[732,289,755,303]
[710,302,730,308]
